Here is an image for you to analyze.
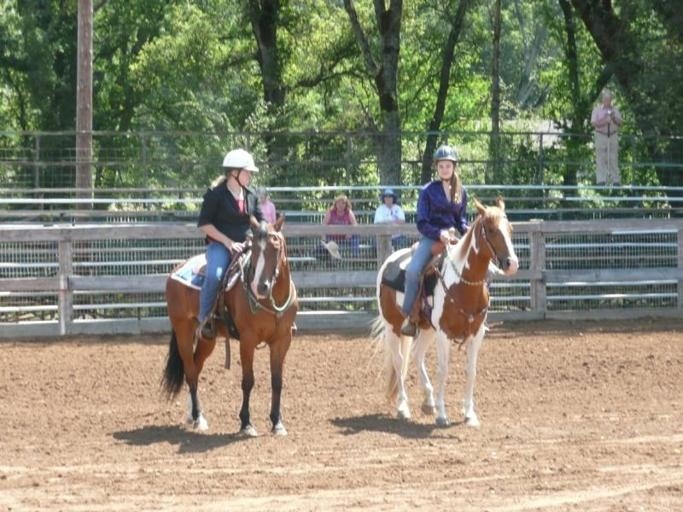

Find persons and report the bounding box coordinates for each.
[254,184,278,225]
[192,148,297,341]
[400,145,472,337]
[589,87,625,189]
[321,193,360,260]
[373,189,406,251]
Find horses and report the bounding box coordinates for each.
[366,194,521,429]
[157,211,299,440]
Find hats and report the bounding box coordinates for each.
[322,240,341,259]
[382,190,397,203]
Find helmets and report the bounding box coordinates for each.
[223,150,259,172]
[435,145,458,161]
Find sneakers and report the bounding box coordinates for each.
[199,321,213,339]
[401,317,416,335]
